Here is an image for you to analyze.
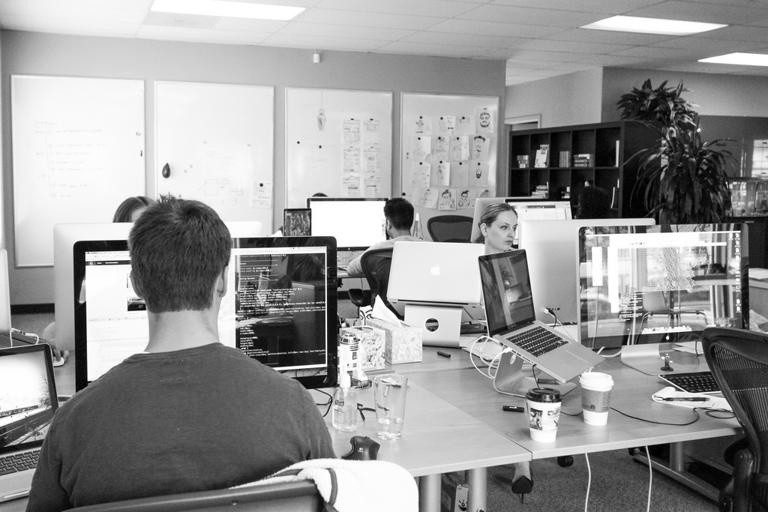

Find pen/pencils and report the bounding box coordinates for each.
[663,397,709,401]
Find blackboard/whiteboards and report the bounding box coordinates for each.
[401,91,497,240]
[155,79,272,236]
[287,89,391,208]
[8,73,146,268]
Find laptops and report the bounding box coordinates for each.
[0,343,59,504]
[477,248,606,384]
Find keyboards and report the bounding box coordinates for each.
[657,367,768,395]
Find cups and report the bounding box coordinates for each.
[331,388,359,434]
[524,387,564,444]
[579,370,613,427]
[373,373,408,440]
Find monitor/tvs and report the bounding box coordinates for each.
[282,207,312,238]
[52,219,263,351]
[517,217,655,327]
[0,248,11,334]
[306,196,390,251]
[503,197,575,249]
[385,239,485,350]
[72,235,340,395]
[469,195,545,243]
[575,221,750,377]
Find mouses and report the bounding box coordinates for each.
[341,435,380,461]
[53,349,65,367]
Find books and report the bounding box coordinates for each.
[516,137,621,210]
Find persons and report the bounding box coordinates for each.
[114,198,152,223]
[473,203,519,257]
[26,197,337,511]
[345,198,423,274]
[573,186,612,220]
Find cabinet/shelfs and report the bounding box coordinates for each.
[508,119,661,222]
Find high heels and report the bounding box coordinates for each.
[512,468,533,502]
